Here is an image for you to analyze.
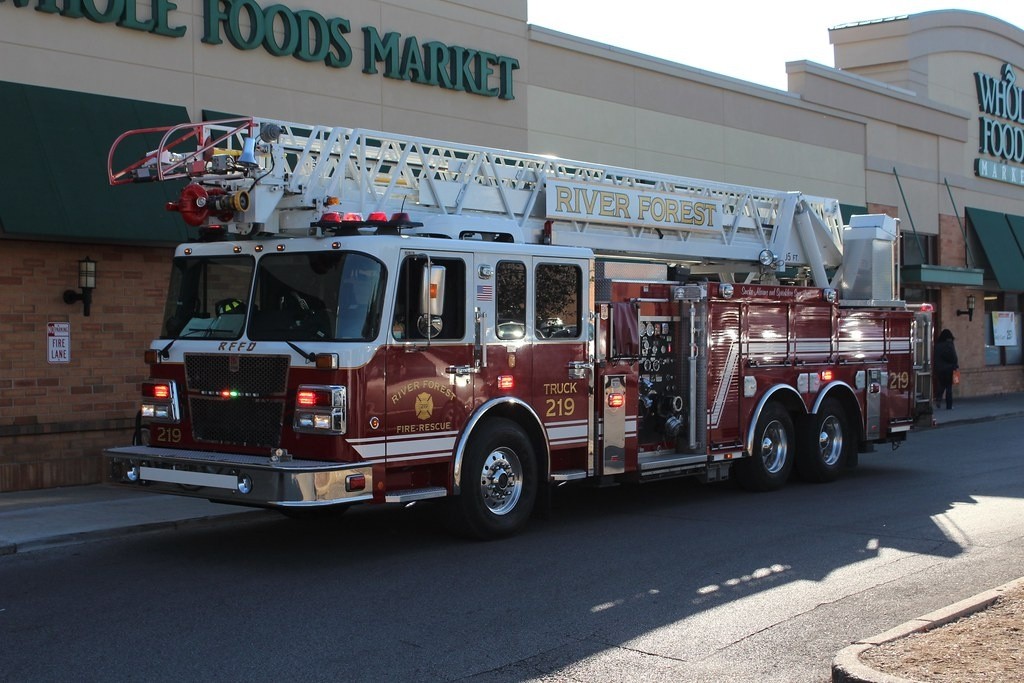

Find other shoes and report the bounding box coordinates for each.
[947,406,952,409]
[936,401,941,408]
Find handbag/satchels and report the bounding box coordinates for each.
[952,370,961,385]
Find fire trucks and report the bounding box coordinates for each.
[100,116,936,542]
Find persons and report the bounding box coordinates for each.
[932,329,959,410]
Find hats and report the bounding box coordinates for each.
[939,329,954,341]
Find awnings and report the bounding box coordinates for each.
[965,206,1024,290]
[0,81,213,244]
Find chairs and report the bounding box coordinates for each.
[261,289,331,338]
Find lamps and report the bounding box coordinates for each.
[957,295,975,321]
[63,255,96,317]
